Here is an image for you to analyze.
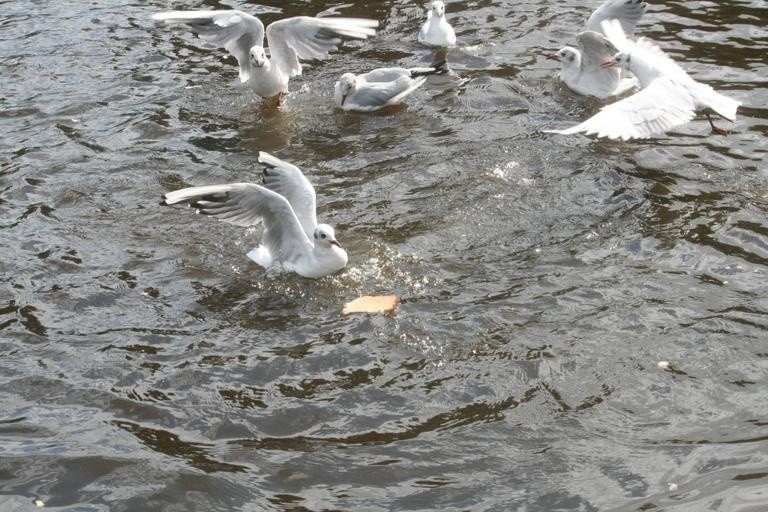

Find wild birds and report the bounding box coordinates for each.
[546,0,649,100]
[151,10,380,109]
[333,67,437,113]
[541,18,742,142]
[158,151,349,280]
[418,0,456,48]
